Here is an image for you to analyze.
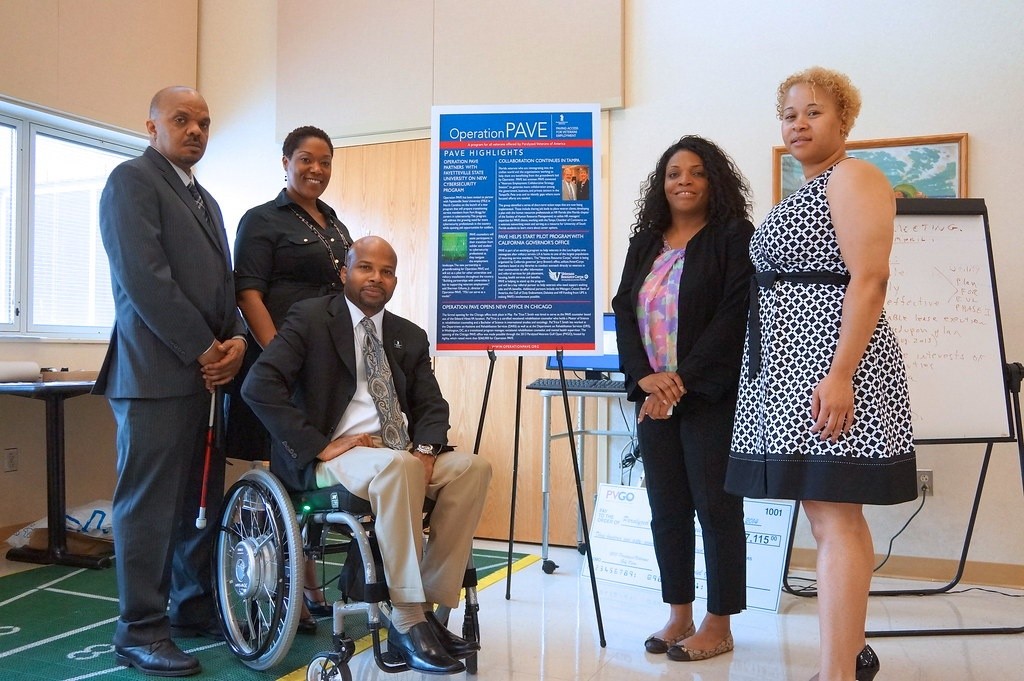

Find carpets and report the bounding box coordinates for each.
[1,548,540,681]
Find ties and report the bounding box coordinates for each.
[189,182,210,225]
[360,317,411,449]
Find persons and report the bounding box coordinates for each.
[240,236,493,675]
[721,64,918,681]
[223,124,355,636]
[91,85,256,677]
[613,135,757,661]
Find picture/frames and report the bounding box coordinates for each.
[772,134,968,210]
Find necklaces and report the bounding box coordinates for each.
[288,204,349,276]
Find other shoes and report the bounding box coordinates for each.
[667,630,733,662]
[645,619,695,652]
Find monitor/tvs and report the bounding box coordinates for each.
[546,313,619,376]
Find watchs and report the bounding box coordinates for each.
[415,443,438,459]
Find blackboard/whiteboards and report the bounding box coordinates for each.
[884,196,1017,444]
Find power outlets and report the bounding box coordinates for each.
[915,468,934,497]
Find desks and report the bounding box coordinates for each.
[0,382,112,571]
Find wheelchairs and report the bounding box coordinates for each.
[211,382,482,681]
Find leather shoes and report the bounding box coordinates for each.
[115,639,201,676]
[171,617,250,641]
[387,620,467,674]
[425,611,479,655]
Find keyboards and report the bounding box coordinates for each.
[526,379,626,392]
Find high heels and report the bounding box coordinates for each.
[854,644,880,681]
[283,600,317,632]
[303,591,333,616]
